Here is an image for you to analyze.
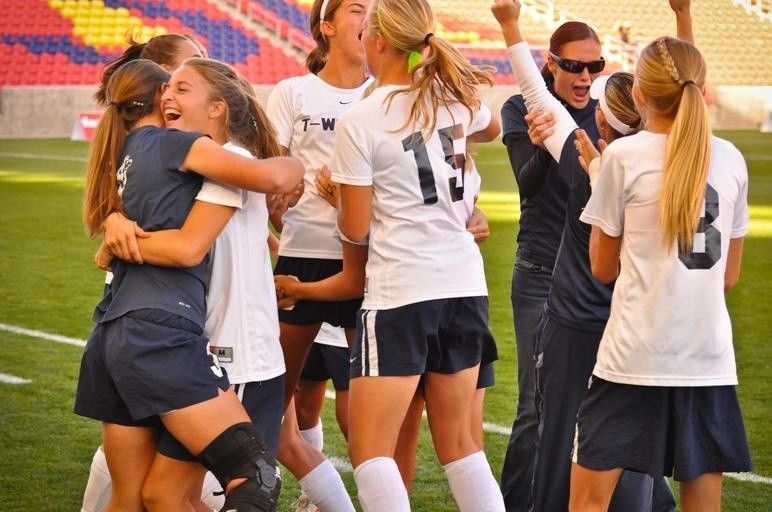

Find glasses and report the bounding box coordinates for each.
[549,52,604,75]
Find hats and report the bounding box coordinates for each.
[589,73,637,136]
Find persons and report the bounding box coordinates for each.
[84,35,225,512]
[314,0,506,512]
[275,79,498,494]
[267,228,350,512]
[491,0,677,512]
[267,0,374,512]
[609,21,637,64]
[500,23,654,512]
[95,59,286,512]
[73,60,304,512]
[569,38,748,512]
